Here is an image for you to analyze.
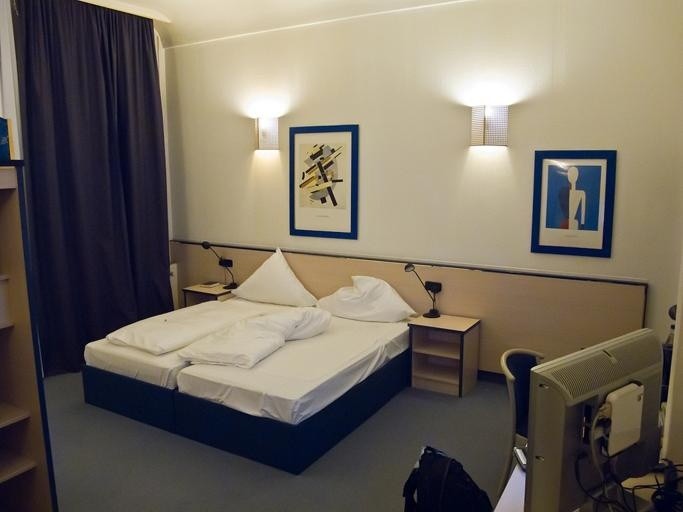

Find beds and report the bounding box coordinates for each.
[83,296,315,434]
[174,316,419,476]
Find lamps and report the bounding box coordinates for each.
[201,241,238,290]
[254,117,279,151]
[404,264,442,318]
[469,104,509,147]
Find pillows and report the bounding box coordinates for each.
[230,248,318,308]
[316,275,417,322]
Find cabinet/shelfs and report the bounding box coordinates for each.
[0,159,59,512]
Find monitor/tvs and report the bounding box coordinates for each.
[524,327,664,512]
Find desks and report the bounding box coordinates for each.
[493,464,683,512]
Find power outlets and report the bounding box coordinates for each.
[219,258,233,267]
[425,281,441,292]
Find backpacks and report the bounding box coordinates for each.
[401,445,493,512]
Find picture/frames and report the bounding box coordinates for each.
[289,123,360,240]
[530,150,617,258]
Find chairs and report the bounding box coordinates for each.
[496,348,544,502]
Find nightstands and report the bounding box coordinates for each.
[181,284,232,307]
[407,313,481,398]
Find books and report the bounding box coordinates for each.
[201,280,220,288]
